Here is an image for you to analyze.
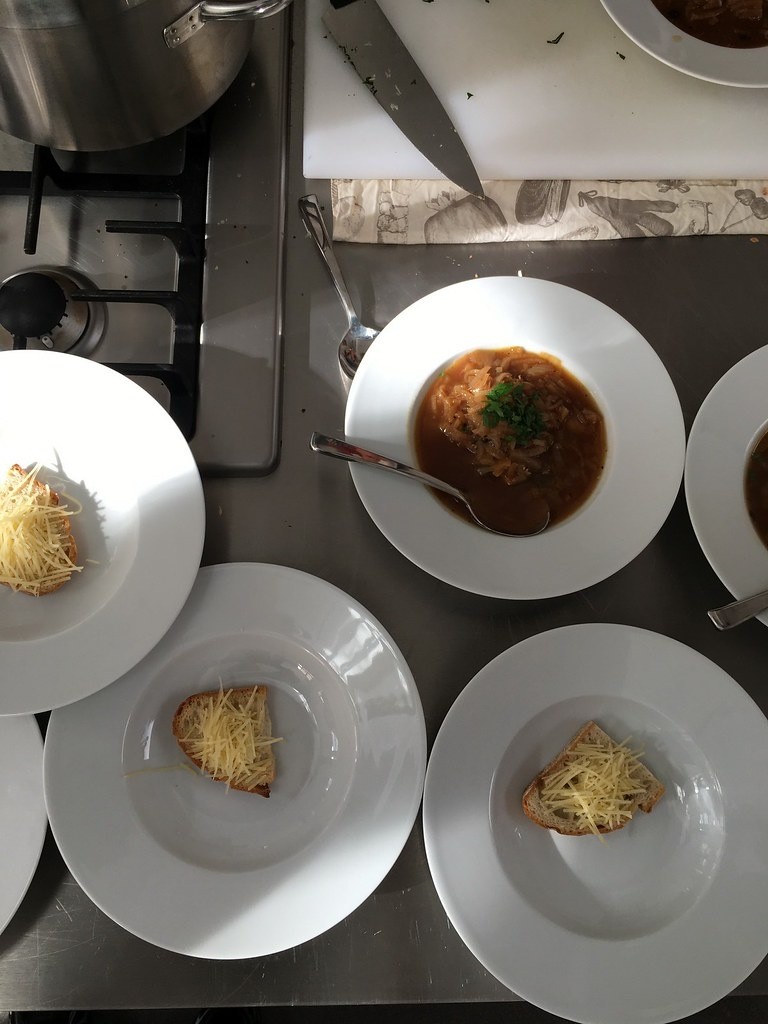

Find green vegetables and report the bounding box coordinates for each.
[478,380,553,445]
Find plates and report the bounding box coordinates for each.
[345,277,686,600]
[686,344,768,627]
[596,0,768,88]
[422,623,768,1024]
[0,350,206,719]
[0,708,48,942]
[42,563,427,960]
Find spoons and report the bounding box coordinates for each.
[310,431,552,540]
[296,195,379,378]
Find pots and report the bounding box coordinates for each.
[0,0,287,152]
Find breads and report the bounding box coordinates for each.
[172,686,275,798]
[522,720,664,836]
[0,463,77,596]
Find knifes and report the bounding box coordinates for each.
[320,0,486,200]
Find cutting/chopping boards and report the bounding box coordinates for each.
[304,0,768,178]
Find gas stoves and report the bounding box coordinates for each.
[0,0,286,478]
